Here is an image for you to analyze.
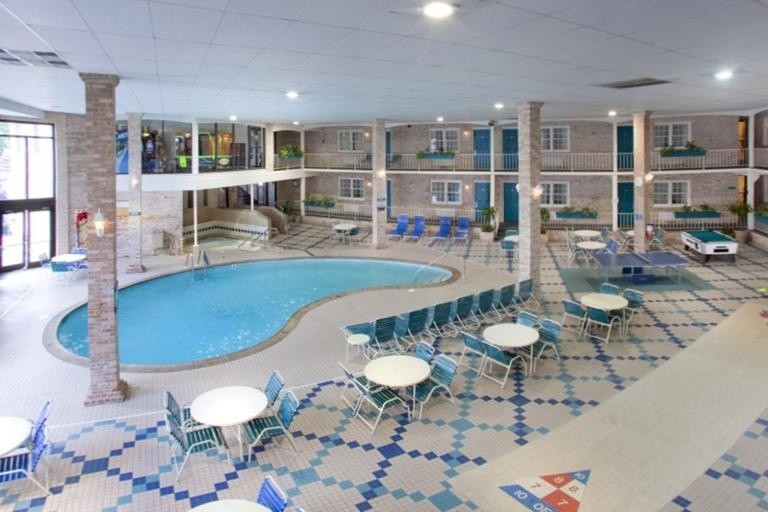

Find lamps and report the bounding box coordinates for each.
[93,207,105,239]
[417,1,460,20]
[645,171,655,185]
[533,182,544,200]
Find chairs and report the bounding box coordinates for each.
[404,215,425,242]
[406,353,458,419]
[500,240,516,263]
[472,289,501,322]
[399,301,458,338]
[345,227,360,245]
[451,293,481,331]
[583,304,621,344]
[514,278,543,311]
[431,216,452,243]
[373,308,434,351]
[163,390,204,454]
[516,311,538,327]
[164,413,231,482]
[600,283,620,295]
[352,377,411,436]
[22,400,51,443]
[623,288,644,335]
[337,360,381,416]
[479,340,528,389]
[559,298,588,337]
[458,330,482,376]
[345,315,400,361]
[388,213,409,240]
[263,369,285,407]
[242,390,300,464]
[0,419,53,495]
[454,216,469,240]
[414,340,435,363]
[520,318,561,375]
[494,283,521,317]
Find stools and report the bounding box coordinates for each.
[345,333,370,364]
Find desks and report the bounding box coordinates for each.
[0,414,32,456]
[577,240,607,250]
[680,229,739,264]
[364,355,430,419]
[50,253,86,265]
[483,322,540,375]
[590,251,690,286]
[580,292,628,334]
[333,224,357,244]
[190,385,268,463]
[575,229,600,240]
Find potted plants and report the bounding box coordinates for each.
[303,197,334,207]
[280,145,304,160]
[415,148,455,159]
[479,208,497,242]
[674,204,721,218]
[539,206,550,241]
[556,206,598,219]
[660,141,706,157]
